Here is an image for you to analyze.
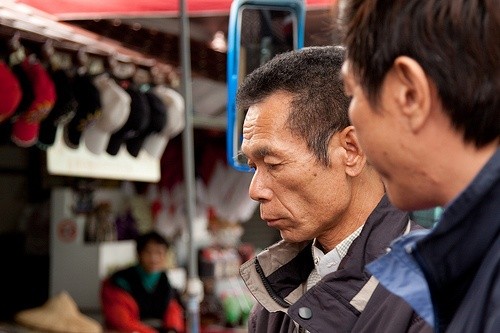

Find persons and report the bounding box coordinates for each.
[101,229,186,333]
[334,0,500,333]
[240,46,429,333]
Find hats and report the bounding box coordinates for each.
[41,66,78,145]
[64,72,103,150]
[85,73,132,155]
[145,85,187,158]
[9,56,56,150]
[0,60,23,123]
[105,86,150,160]
[14,289,102,333]
[126,92,167,158]
